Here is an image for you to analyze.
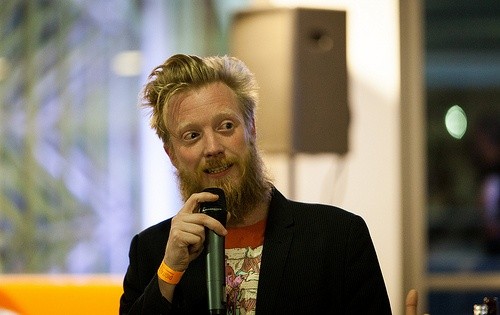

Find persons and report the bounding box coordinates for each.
[118,54,430,315]
[468,113,500,259]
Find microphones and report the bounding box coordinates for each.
[200,188,227,315]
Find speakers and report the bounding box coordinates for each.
[230,7,349,157]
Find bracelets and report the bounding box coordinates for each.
[157,260,185,285]
